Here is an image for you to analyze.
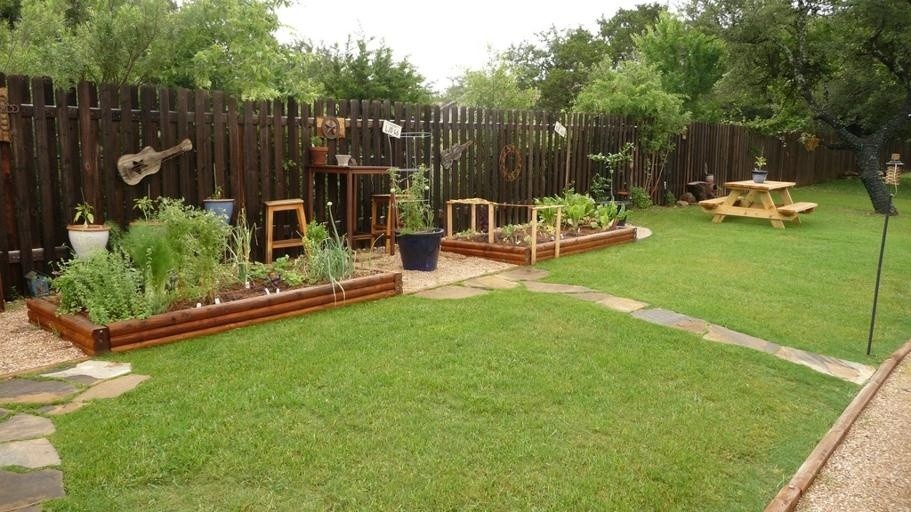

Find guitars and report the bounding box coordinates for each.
[118,139,193,186]
[440,140,473,170]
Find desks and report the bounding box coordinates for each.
[306,164,395,256]
[698,179,820,230]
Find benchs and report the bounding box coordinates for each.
[778,198,819,216]
[697,194,743,210]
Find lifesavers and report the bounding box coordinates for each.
[499,144,522,182]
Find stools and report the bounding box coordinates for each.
[264,196,308,263]
[369,191,412,255]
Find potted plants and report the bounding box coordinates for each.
[203,181,235,226]
[66,197,111,254]
[309,134,330,165]
[386,163,447,272]
[752,156,771,183]
[129,195,163,235]
